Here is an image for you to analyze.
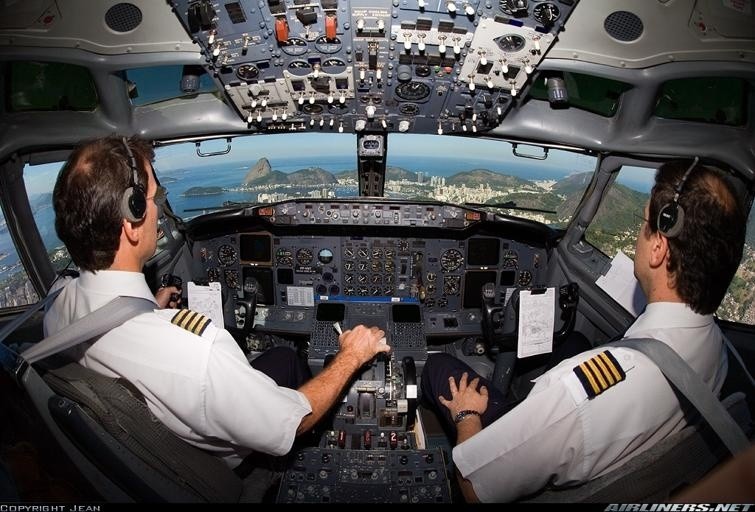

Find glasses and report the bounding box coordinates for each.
[144,186,169,206]
[633,209,650,226]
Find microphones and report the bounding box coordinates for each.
[120,135,148,223]
[656,154,701,238]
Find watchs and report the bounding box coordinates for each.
[453,410,481,426]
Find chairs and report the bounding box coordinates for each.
[2,342,273,512]
[514,389,754,512]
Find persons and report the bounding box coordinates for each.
[43,137,391,480]
[421,158,748,503]
